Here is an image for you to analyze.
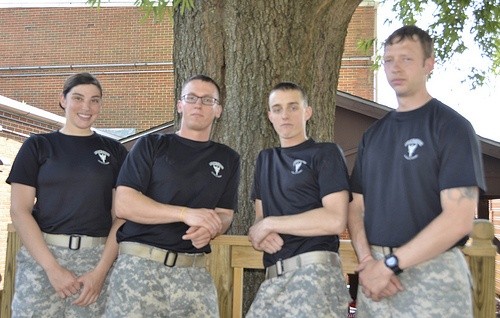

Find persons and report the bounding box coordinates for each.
[6,72,129,318]
[246,82,354,318]
[346,26,488,318]
[102,74,239,318]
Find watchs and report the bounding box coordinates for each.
[385,254,403,275]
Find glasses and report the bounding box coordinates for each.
[180,94,220,106]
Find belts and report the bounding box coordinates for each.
[371,244,398,256]
[265,250,341,279]
[41,230,109,251]
[118,242,208,268]
